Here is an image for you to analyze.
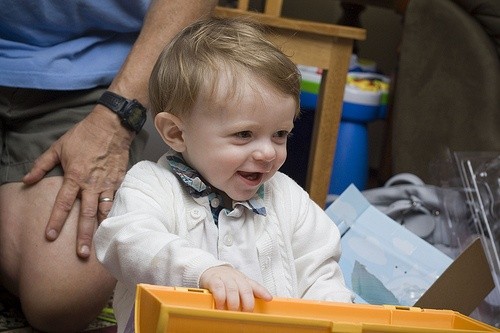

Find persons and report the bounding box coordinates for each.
[0,0,221,332]
[92,17,356,333]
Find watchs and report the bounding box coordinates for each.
[95,91,147,135]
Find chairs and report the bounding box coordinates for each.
[210,0,368,212]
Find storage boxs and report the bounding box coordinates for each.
[120,281,499,332]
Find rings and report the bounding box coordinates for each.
[98,197,114,203]
